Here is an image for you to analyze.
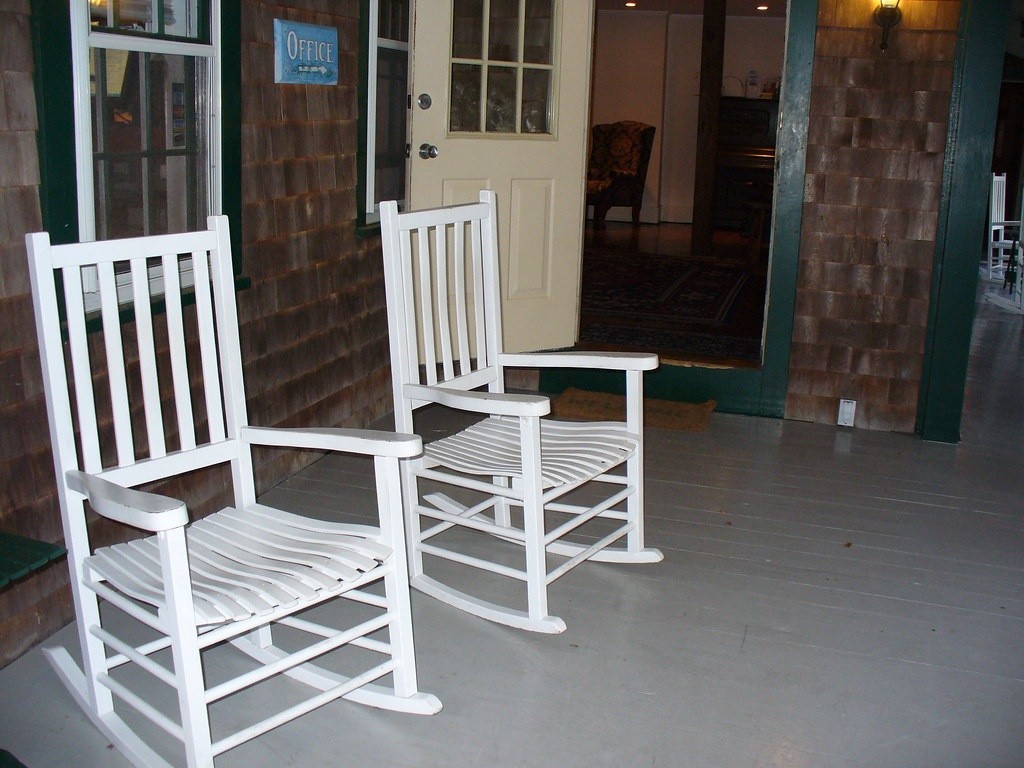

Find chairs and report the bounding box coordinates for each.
[379,190,664,635]
[26,215,443,768]
[987,173,1022,280]
[585,120,657,230]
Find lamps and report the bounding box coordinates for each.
[873,0,902,56]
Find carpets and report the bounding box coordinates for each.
[579,322,761,362]
[583,245,754,326]
[547,383,716,431]
